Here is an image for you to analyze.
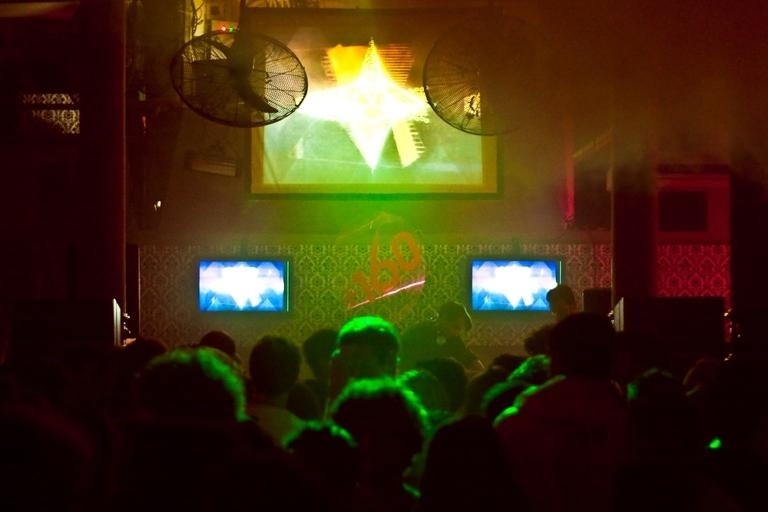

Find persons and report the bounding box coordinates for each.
[2,313,768,510]
[401,302,476,368]
[546,284,576,322]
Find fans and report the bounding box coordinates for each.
[167,23,309,180]
[423,9,564,138]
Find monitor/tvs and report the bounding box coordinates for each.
[468,256,564,317]
[197,258,288,313]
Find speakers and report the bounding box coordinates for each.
[613,297,723,343]
[11,298,121,412]
